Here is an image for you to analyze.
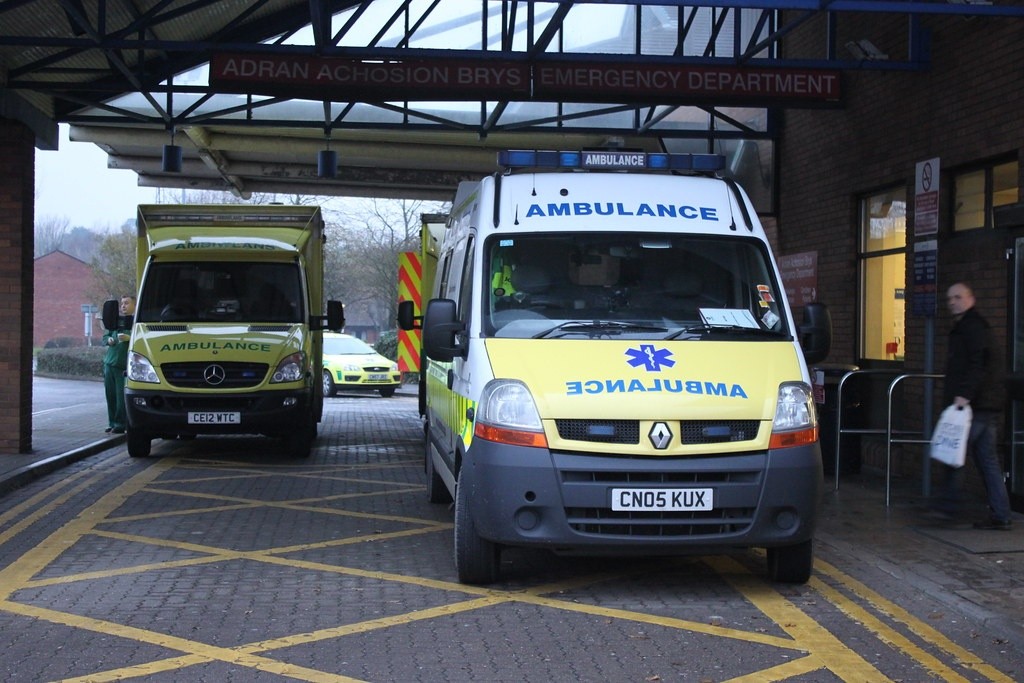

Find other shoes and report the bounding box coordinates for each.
[974,512,1013,530]
[105,428,111,432]
[111,429,124,433]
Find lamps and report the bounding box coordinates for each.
[845,38,888,61]
[163,136,181,173]
[316,131,336,178]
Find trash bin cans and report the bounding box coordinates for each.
[809,361,862,473]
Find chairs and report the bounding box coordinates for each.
[497,263,573,317]
[659,265,722,318]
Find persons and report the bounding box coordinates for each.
[102,296,136,433]
[922,283,1014,530]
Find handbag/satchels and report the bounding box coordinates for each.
[931,404,972,467]
